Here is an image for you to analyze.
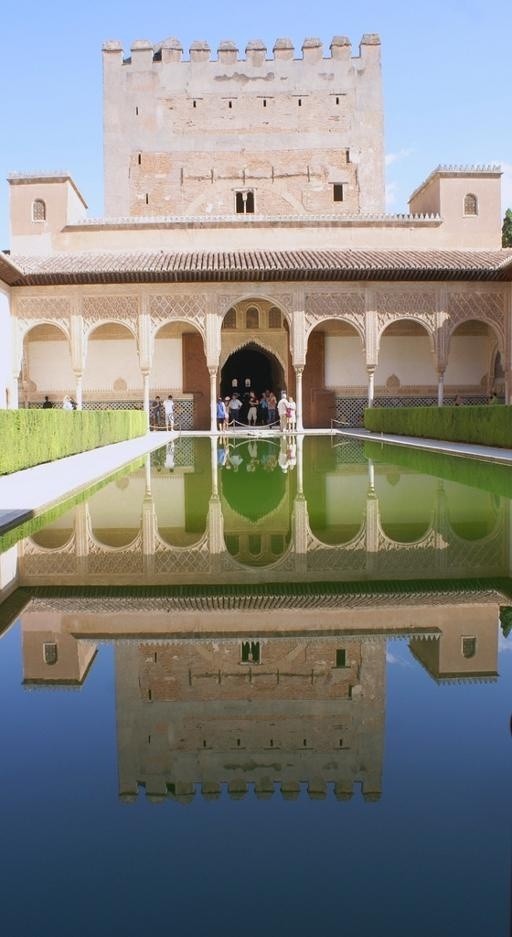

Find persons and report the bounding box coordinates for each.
[62,395,73,410]
[278,434,289,473]
[259,390,278,425]
[152,449,162,471]
[163,394,176,432]
[276,392,290,432]
[260,437,277,472]
[488,391,499,405]
[228,391,244,427]
[453,394,464,406]
[287,435,297,471]
[69,395,78,410]
[165,439,177,474]
[247,391,259,426]
[287,395,296,432]
[244,437,260,472]
[222,436,232,470]
[228,438,244,472]
[216,394,226,432]
[151,394,163,431]
[42,395,53,408]
[223,394,231,430]
[218,435,224,471]
